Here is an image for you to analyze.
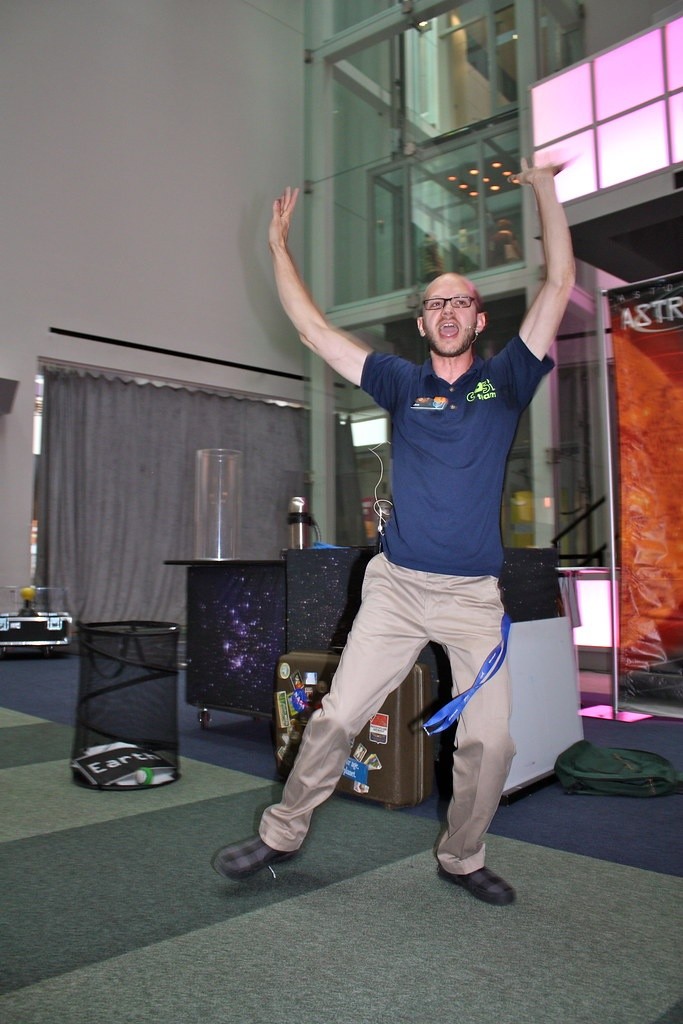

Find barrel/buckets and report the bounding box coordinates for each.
[195,448,244,560]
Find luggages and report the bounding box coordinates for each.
[275,648,433,810]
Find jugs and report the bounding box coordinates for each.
[288,497,321,549]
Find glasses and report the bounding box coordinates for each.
[423,296,482,310]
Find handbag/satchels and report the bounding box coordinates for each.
[554,740,683,798]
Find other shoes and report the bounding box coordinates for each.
[213,834,299,882]
[437,864,514,906]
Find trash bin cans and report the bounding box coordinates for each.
[70,619,181,791]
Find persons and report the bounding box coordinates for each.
[212,157,575,908]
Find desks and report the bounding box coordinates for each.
[164,559,285,736]
[283,546,565,800]
